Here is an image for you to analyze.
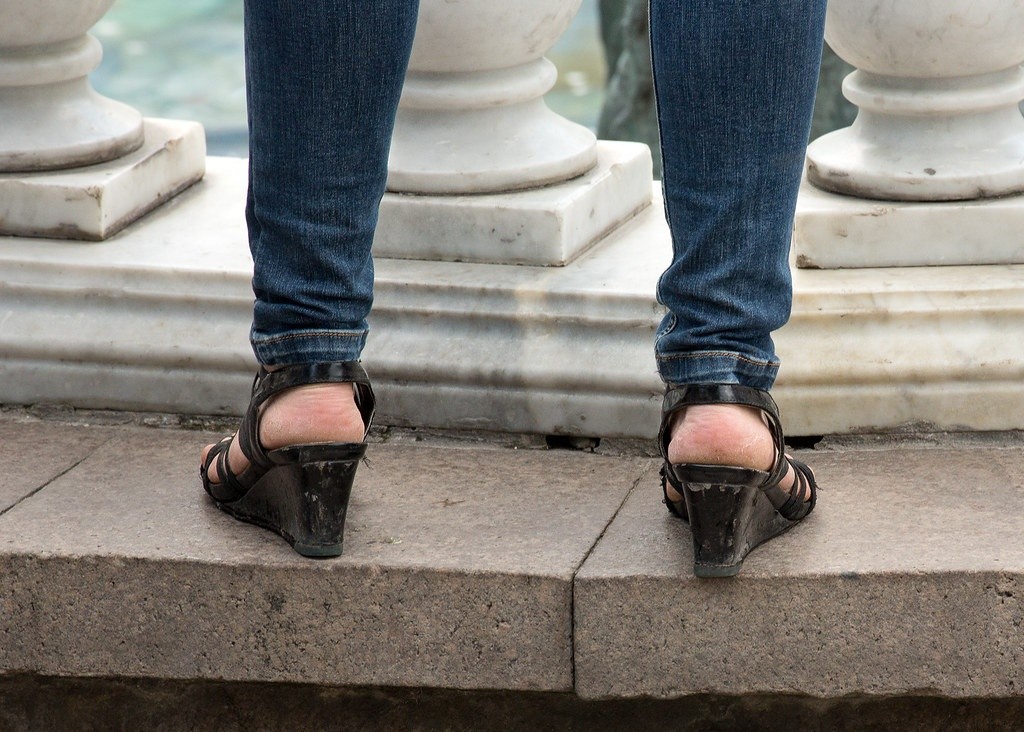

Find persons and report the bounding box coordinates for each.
[198,0,831,578]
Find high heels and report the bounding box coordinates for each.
[199,357,376,558]
[656,384,817,578]
